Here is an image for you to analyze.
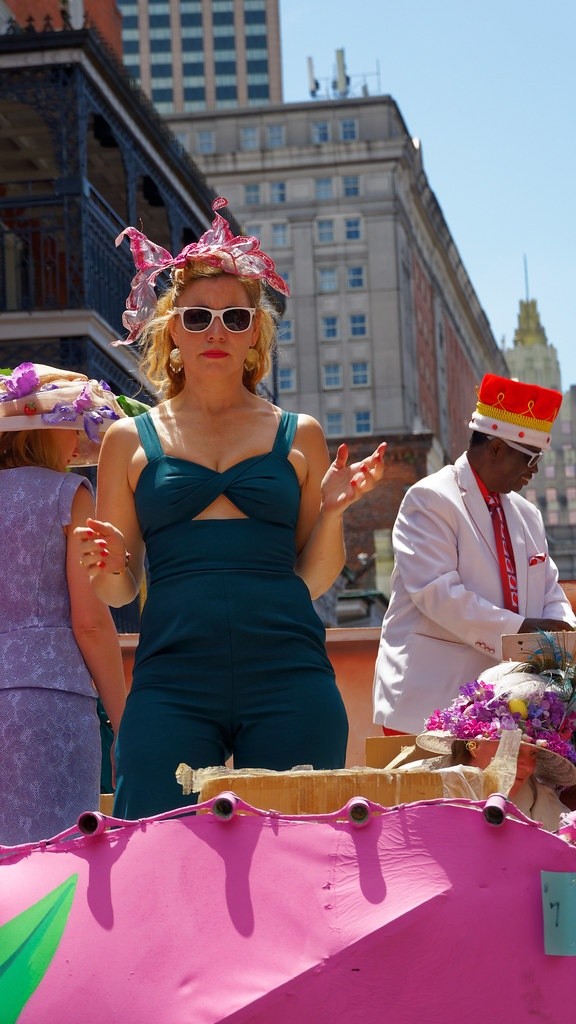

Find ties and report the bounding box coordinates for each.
[485,494,519,615]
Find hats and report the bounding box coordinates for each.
[0,362,152,468]
[468,373,562,450]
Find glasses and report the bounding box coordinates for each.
[487,436,543,469]
[173,307,256,333]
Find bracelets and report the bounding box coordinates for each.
[110,550,132,575]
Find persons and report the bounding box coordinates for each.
[72,198,387,825]
[0,360,127,850]
[373,372,576,735]
